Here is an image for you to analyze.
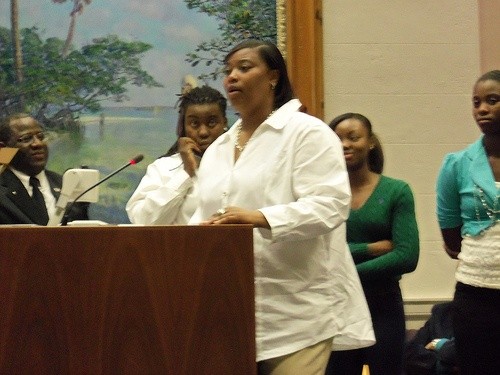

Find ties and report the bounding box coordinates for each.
[29,176,49,226]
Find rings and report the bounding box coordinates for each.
[217,209,225,214]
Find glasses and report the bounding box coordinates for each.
[4,130,51,145]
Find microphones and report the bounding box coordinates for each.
[60,154,144,226]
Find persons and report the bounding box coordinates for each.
[436,70,500,375]
[0,112,89,226]
[187,39,376,375]
[325,112,420,375]
[125,85,228,225]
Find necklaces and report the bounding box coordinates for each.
[235,108,278,152]
